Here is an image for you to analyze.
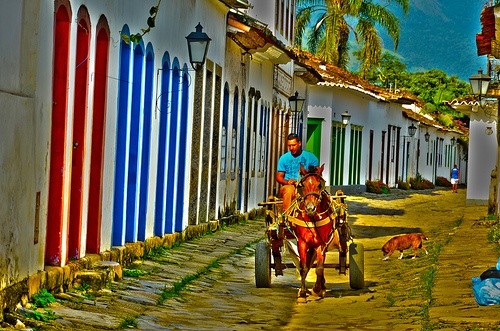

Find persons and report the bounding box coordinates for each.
[450,164,460,193]
[274,133,320,215]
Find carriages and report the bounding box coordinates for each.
[255,162,365,304]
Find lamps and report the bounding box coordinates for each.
[469,67,498,123]
[276,91,305,138]
[155,23,212,121]
[425,132,430,142]
[341,111,351,127]
[401,124,417,137]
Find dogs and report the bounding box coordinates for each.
[382,233,428,261]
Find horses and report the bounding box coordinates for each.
[294,163,335,305]
